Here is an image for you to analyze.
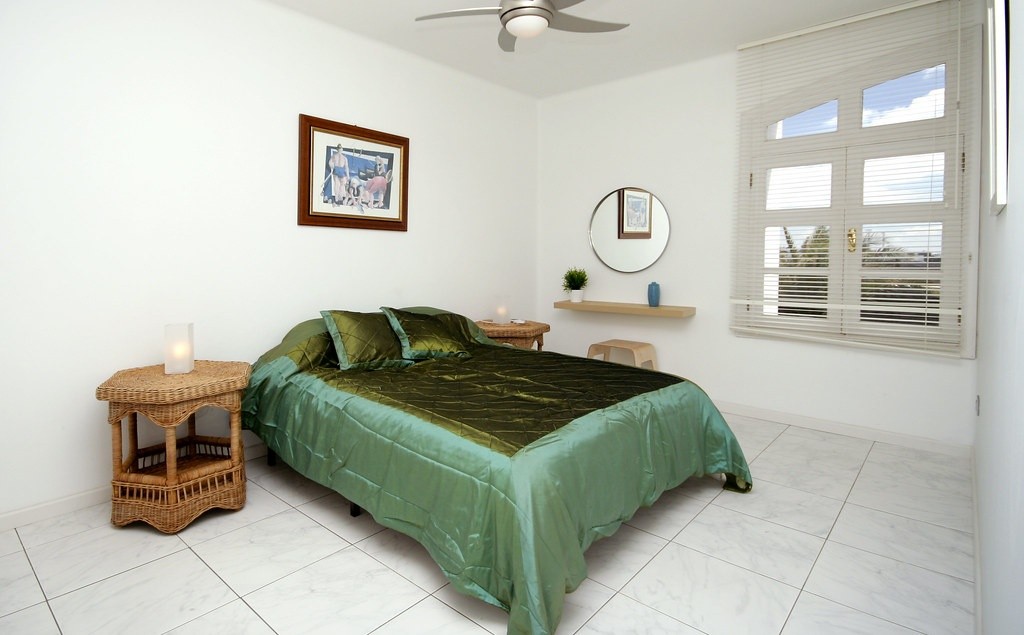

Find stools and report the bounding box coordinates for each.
[586,339,658,370]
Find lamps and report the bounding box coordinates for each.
[163,322,195,375]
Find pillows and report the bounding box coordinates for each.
[320,310,416,372]
[379,306,475,359]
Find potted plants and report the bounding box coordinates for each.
[562,268,588,302]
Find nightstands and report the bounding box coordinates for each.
[96,359,252,535]
[474,320,549,351]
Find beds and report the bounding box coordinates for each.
[229,305,752,635]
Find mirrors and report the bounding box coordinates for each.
[589,188,671,274]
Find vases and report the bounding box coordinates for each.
[647,281,660,307]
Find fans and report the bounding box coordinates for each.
[415,0,629,53]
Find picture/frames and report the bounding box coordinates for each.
[618,189,652,239]
[298,114,410,233]
